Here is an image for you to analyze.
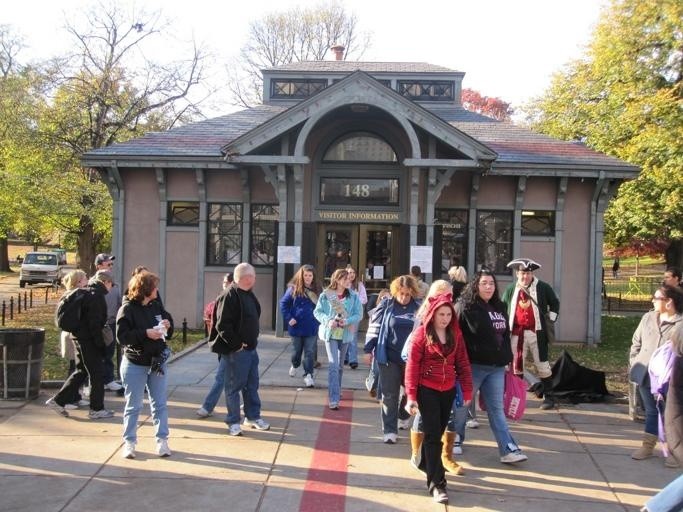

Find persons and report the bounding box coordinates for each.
[501,258,561,410]
[124,266,163,393]
[207,263,272,435]
[627,265,683,512]
[198,272,244,417]
[61,269,90,408]
[114,271,173,459]
[83,253,123,396]
[613,261,619,278]
[281,263,367,410]
[46,269,115,419]
[363,265,528,502]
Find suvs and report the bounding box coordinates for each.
[16,251,60,288]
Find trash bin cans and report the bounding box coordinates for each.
[0,327,46,400]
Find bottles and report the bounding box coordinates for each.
[409,407,419,416]
[335,313,343,327]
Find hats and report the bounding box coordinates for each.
[94,253,115,265]
[96,269,117,287]
[507,258,541,271]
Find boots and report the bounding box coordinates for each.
[632,432,679,467]
[539,375,554,409]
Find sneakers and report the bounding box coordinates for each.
[196,408,213,416]
[350,363,357,368]
[124,444,135,458]
[45,382,123,418]
[230,404,270,436]
[289,361,321,387]
[157,441,171,456]
[329,402,339,409]
[500,453,528,463]
[384,419,478,503]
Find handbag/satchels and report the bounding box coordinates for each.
[503,368,528,419]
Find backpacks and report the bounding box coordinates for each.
[58,288,95,333]
